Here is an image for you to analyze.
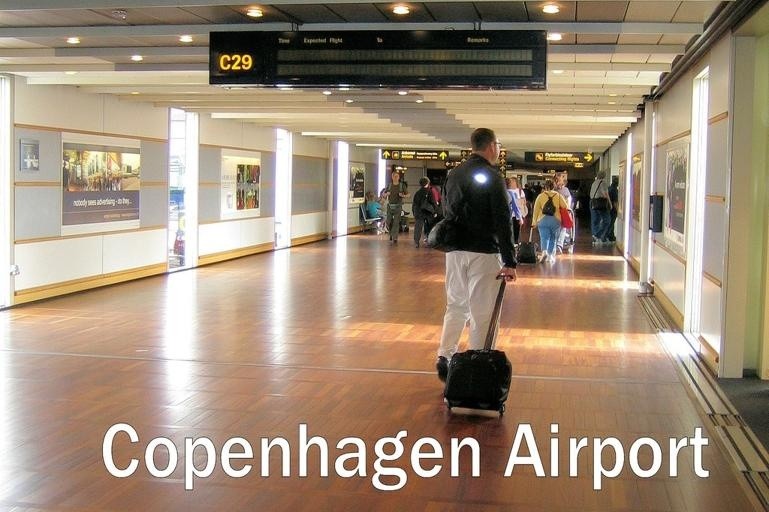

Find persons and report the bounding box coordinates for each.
[428,127,518,382]
[358,167,619,266]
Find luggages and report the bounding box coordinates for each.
[443,346,512,415]
[516,241,539,265]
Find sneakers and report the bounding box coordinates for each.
[557,245,563,255]
[387,237,398,244]
[540,252,555,264]
[415,241,420,248]
[592,235,616,244]
[377,226,389,234]
[435,355,448,383]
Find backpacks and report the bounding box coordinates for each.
[540,191,557,216]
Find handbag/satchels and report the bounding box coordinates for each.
[591,197,609,211]
[421,200,441,216]
[560,206,573,228]
[427,217,462,253]
[389,204,401,210]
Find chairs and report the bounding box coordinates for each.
[360,203,384,234]
